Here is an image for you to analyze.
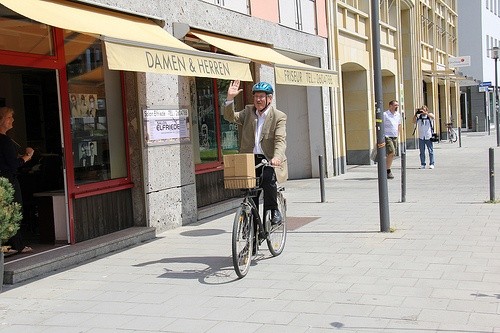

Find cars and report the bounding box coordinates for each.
[72,123,108,151]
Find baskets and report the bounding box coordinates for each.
[223,174,260,190]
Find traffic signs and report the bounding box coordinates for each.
[479,82,492,86]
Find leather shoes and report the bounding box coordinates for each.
[271,210,282,224]
[239,242,256,258]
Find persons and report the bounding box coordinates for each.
[79,143,97,167]
[223,80,288,259]
[383,100,402,179]
[413,106,434,169]
[71,95,98,118]
[0,106,34,257]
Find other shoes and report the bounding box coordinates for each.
[387,169,395,178]
[430,165,434,168]
[4,249,18,256]
[419,165,425,169]
[21,246,34,252]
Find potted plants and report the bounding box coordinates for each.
[0,176,22,287]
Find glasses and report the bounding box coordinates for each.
[253,94,270,100]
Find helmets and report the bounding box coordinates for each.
[252,82,273,94]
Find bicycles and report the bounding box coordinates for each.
[443,121,457,143]
[224,159,287,278]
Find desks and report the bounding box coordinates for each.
[33,190,69,243]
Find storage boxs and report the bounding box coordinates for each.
[224,154,257,189]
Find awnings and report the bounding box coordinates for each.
[0,0,253,83]
[187,29,340,88]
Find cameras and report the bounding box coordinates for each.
[416,109,423,114]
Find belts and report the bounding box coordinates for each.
[255,154,265,158]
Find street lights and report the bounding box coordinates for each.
[491,46,500,147]
